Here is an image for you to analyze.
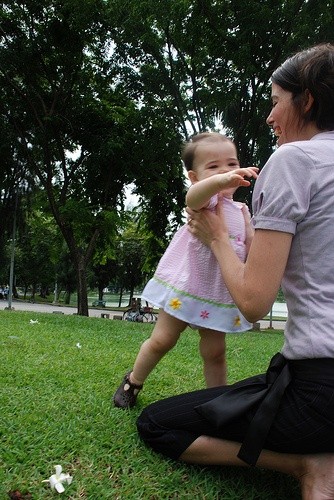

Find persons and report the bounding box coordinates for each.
[113,133,259,409]
[135,42,334,500]
[0,286,9,300]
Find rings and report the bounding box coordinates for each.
[186,219,193,224]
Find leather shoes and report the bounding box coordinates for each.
[114,370,143,410]
